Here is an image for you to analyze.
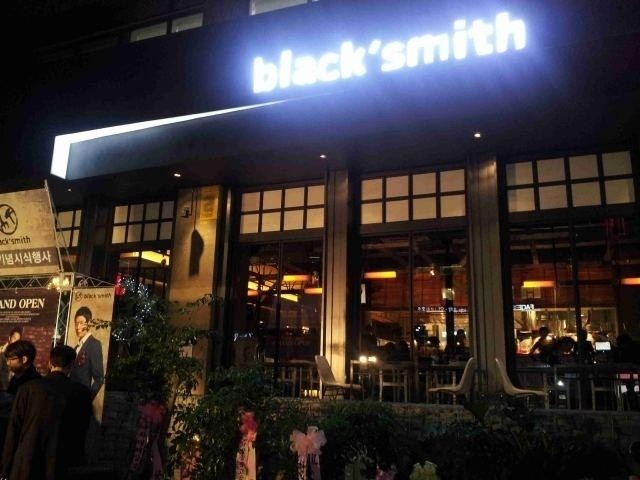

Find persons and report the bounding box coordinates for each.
[0,345,92,480]
[0,327,23,383]
[66,306,104,402]
[528,326,640,361]
[386,327,466,360]
[4,339,43,394]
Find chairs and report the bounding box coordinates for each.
[428,357,594,411]
[379,360,411,403]
[313,355,362,400]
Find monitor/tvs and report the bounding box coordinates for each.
[595,342,612,351]
